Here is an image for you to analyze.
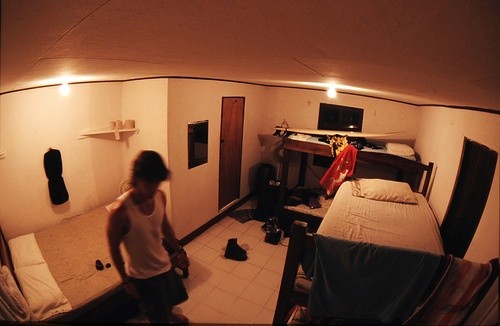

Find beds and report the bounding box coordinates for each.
[273,130,500,326]
[0,190,138,326]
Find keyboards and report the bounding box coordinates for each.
[319,136,364,150]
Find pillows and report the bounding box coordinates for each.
[8,233,46,268]
[14,264,68,318]
[354,175,418,204]
[386,142,414,156]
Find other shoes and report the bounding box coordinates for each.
[225,238,247,261]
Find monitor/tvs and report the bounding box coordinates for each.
[316,103,364,132]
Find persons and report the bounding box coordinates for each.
[106,151,188,326]
